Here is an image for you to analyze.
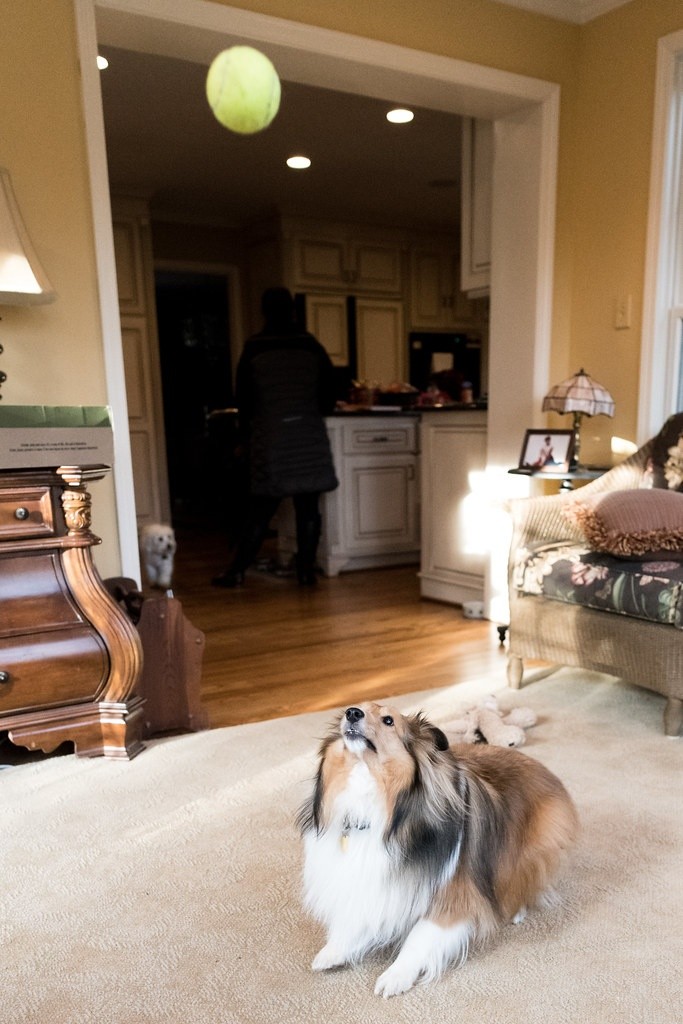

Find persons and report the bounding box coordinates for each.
[525,437,554,470]
[212,286,338,587]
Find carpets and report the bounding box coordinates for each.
[0,667,683,1024]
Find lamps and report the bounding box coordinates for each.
[539,368,615,476]
[0,167,60,401]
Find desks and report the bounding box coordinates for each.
[485,473,592,647]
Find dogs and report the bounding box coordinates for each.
[295,700,577,999]
[141,522,179,588]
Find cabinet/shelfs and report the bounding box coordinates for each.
[0,464,153,765]
[289,412,489,612]
[274,112,489,389]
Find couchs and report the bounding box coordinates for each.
[507,411,683,736]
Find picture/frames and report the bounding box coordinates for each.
[519,428,576,473]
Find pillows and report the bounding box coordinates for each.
[561,486,683,561]
